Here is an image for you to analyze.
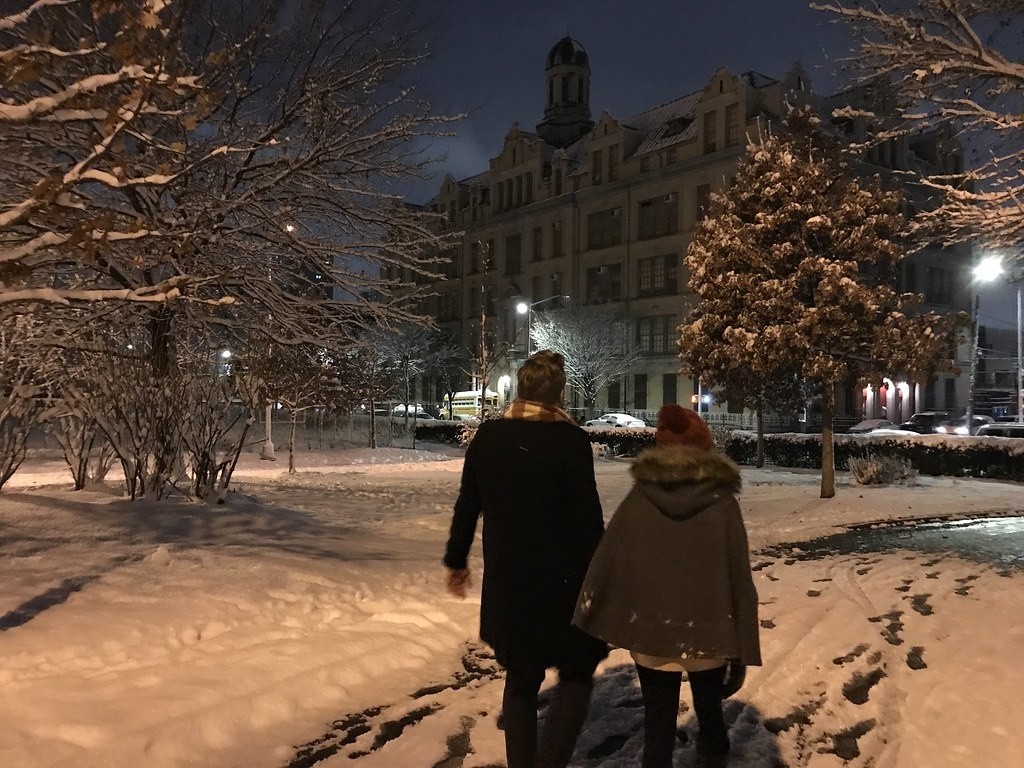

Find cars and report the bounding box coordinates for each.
[938,414,1024,437]
[350,396,433,419]
[849,419,898,434]
[585,413,646,428]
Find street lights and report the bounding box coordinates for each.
[691,377,711,418]
[529,295,570,358]
[261,224,294,461]
[965,257,1004,437]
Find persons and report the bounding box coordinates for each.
[442,350,605,768]
[571,403,763,768]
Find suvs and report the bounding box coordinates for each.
[899,411,949,434]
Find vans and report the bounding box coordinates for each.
[438,390,499,421]
